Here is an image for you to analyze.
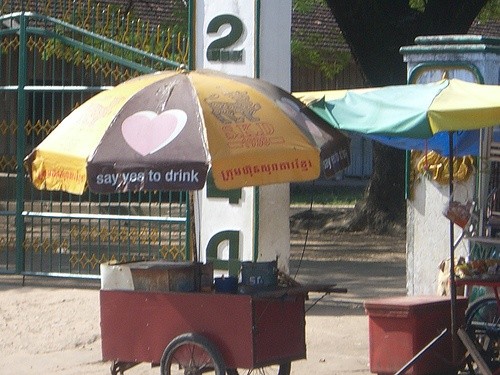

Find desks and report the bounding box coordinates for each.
[364,295,469,375]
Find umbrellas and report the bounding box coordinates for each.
[22,62,352,293]
[292,70,500,362]
